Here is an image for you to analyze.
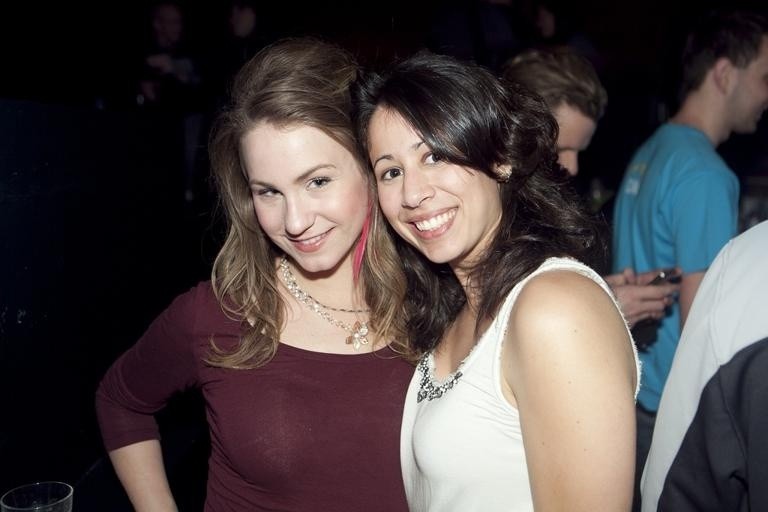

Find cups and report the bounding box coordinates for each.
[0,480,77,511]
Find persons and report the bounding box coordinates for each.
[89,39,428,512]
[498,41,681,347]
[123,4,197,109]
[614,214,768,511]
[609,1,767,512]
[346,43,643,512]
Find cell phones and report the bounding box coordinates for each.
[643,265,676,288]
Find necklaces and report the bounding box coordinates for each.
[407,336,469,403]
[278,254,398,355]
